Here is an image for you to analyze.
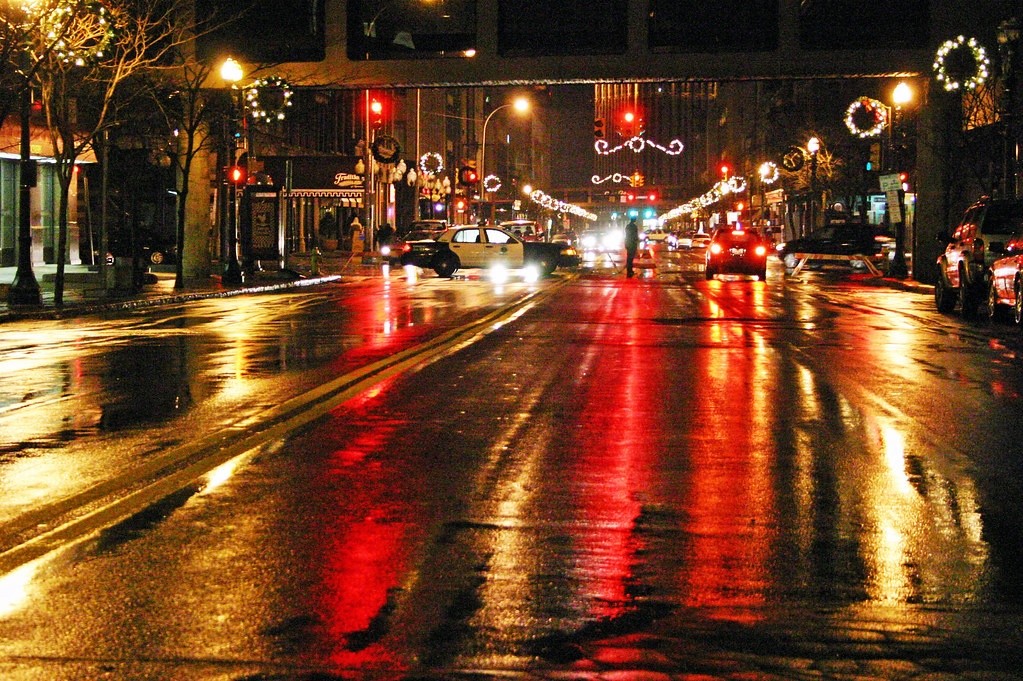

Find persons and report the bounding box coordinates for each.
[343,207,365,251]
[880,214,892,233]
[625,216,639,279]
[556,225,564,234]
[319,211,341,240]
[376,224,394,250]
[524,227,534,236]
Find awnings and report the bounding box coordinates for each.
[752,207,770,218]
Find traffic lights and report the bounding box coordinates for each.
[648,192,659,202]
[643,207,654,219]
[594,118,606,138]
[626,192,638,205]
[621,111,646,138]
[628,209,636,218]
[371,100,383,131]
[718,164,728,182]
[866,143,880,172]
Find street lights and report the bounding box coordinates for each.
[221,58,244,285]
[890,81,912,278]
[809,136,820,228]
[480,100,529,221]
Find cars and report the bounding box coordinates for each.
[382,218,582,277]
[986,223,1023,327]
[577,226,711,252]
[703,229,768,281]
[776,224,892,270]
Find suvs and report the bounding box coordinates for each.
[935,195,1023,315]
